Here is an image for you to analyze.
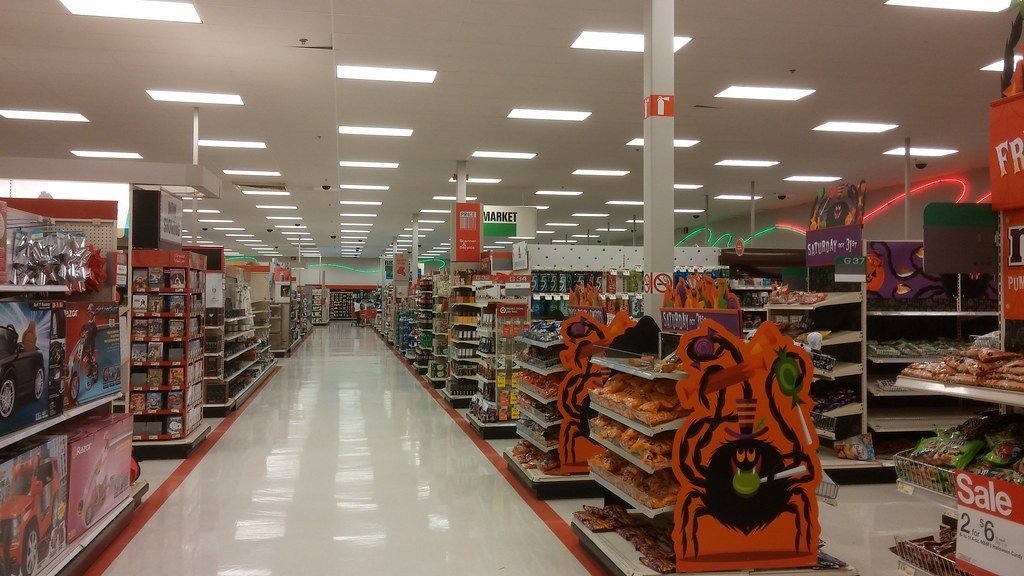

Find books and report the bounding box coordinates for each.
[129,391,184,409]
[132,266,185,288]
[149,367,182,386]
[130,294,185,314]
[187,270,204,433]
[130,343,163,363]
[131,318,185,338]
[167,417,184,432]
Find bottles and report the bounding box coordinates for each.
[418,312,432,329]
[418,293,432,308]
[415,348,429,365]
[418,274,433,290]
[419,332,432,347]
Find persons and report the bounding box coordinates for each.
[91,431,110,503]
[77,304,98,378]
[353,298,361,327]
